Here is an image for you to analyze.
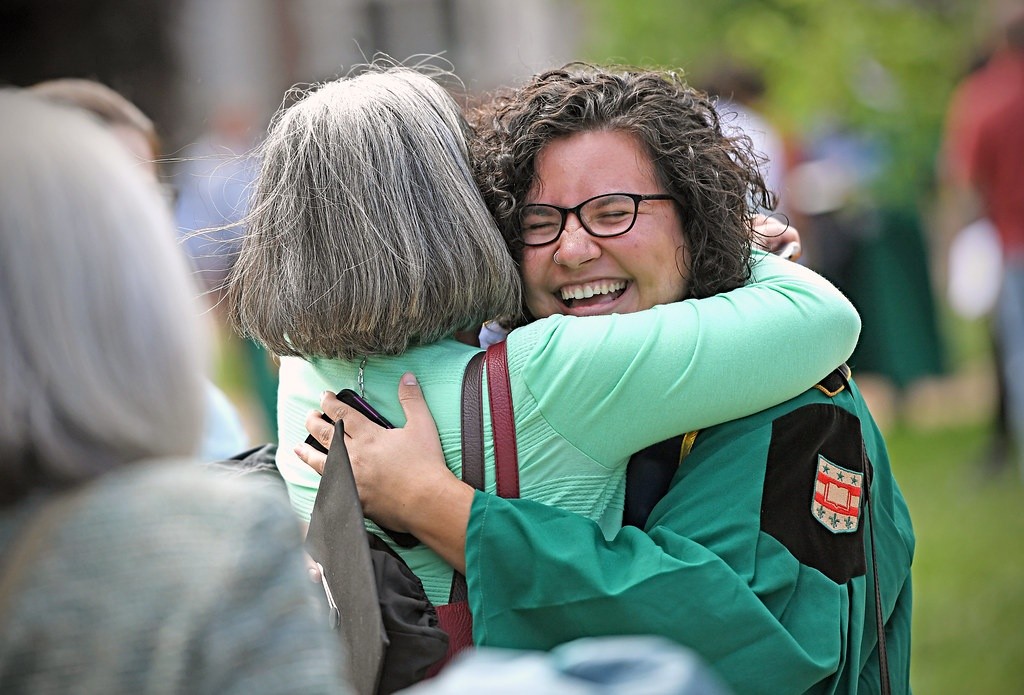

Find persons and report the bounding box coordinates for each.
[0,79,358,695]
[224,67,862,695]
[691,1,1024,480]
[295,63,915,695]
[170,106,265,282]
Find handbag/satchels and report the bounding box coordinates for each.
[423,340,521,679]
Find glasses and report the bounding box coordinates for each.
[515,193,674,247]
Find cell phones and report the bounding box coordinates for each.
[772,242,801,261]
[304,388,420,550]
